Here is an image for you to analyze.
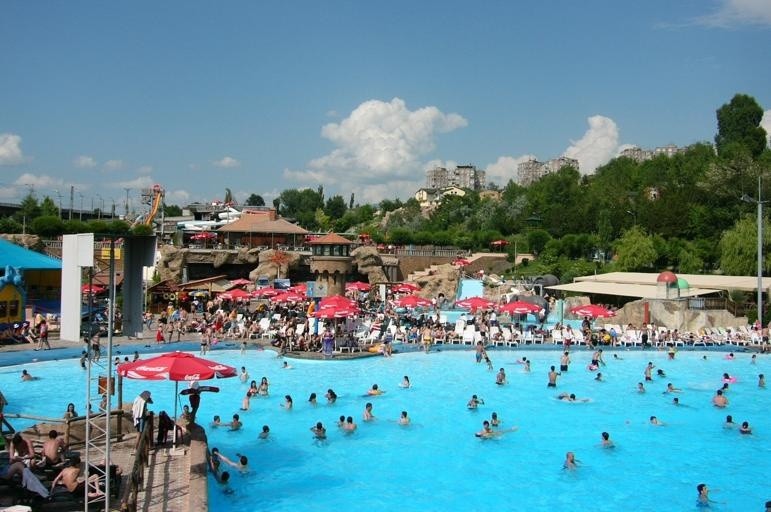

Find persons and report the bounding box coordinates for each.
[0,237,770,512]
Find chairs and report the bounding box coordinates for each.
[0,425,122,511]
[209,304,771,356]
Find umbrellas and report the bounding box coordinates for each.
[190,233,217,249]
